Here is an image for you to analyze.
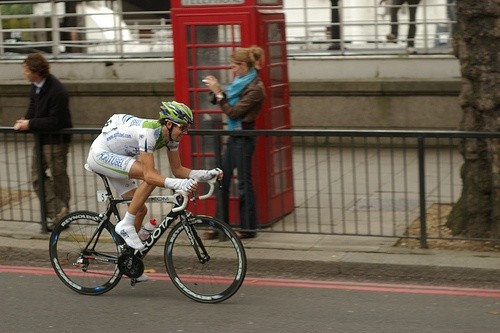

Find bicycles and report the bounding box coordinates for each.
[47,162,248,303]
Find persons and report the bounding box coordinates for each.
[14,53,74,232]
[87,101,223,281]
[202,45,266,240]
[379,0,421,54]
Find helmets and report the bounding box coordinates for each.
[157,100,193,128]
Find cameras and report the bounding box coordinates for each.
[202,79,209,86]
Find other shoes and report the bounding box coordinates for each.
[135,273,149,282]
[115,220,144,250]
[234,230,258,239]
[202,229,220,240]
[46,207,70,230]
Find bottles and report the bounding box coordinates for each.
[138,219,158,241]
